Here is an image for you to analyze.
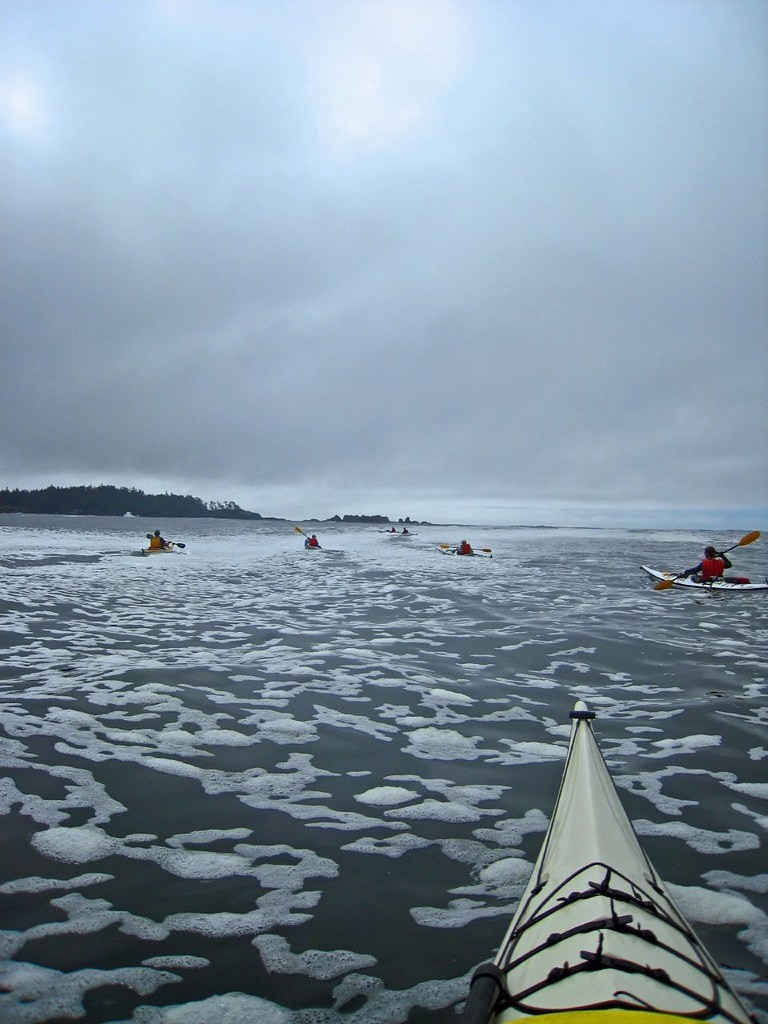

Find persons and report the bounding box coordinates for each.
[391,527,396,532]
[307,535,318,547]
[684,546,733,578]
[403,527,408,534]
[150,530,169,549]
[456,540,473,555]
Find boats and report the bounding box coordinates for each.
[141,547,188,555]
[435,546,493,559]
[305,542,321,550]
[639,564,768,593]
[378,529,418,536]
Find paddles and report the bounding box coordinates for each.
[295,526,323,549]
[146,533,185,548]
[440,545,492,552]
[654,530,760,589]
